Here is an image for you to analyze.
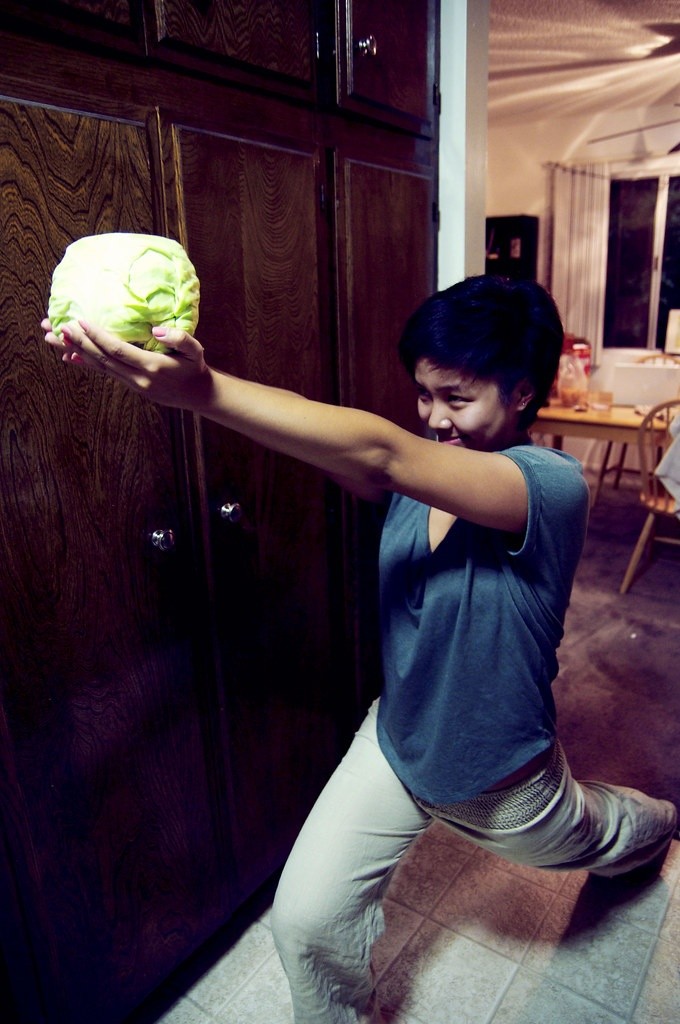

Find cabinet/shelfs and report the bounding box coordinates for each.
[484,212,539,279]
[0,0,443,1024]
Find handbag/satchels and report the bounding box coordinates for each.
[558,354,587,409]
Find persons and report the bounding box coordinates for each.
[42,273,677,1023]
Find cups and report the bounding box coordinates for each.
[572,392,589,411]
[600,393,613,411]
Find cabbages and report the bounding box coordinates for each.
[48,231,201,354]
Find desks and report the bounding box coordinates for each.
[531,404,672,508]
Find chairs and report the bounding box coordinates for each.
[619,396,680,597]
